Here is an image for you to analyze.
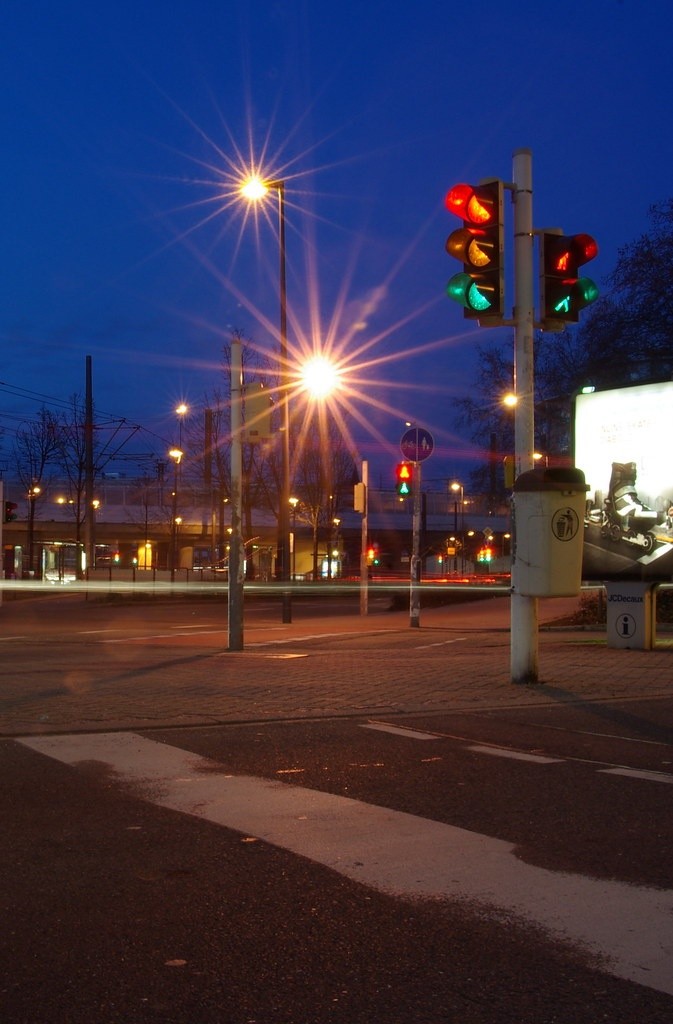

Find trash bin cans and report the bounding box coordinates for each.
[512,467,591,598]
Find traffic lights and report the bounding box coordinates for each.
[2,499,18,524]
[485,548,491,563]
[443,179,505,322]
[394,461,413,498]
[368,548,378,565]
[540,232,598,327]
[479,549,485,563]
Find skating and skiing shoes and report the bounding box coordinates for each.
[599,462,665,555]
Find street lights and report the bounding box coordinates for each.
[169,404,187,584]
[28,487,39,574]
[500,532,510,575]
[289,498,299,583]
[451,482,466,576]
[241,177,292,622]
